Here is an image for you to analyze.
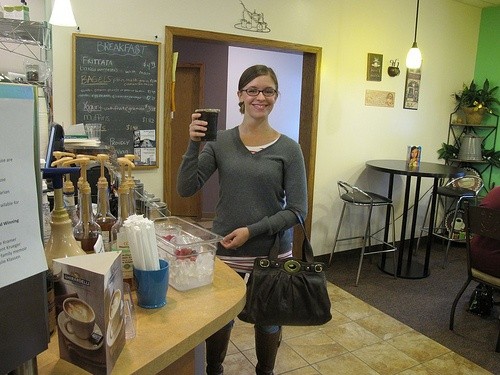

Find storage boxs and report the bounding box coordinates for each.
[4,6,30,21]
[152,217,224,293]
[45,168,112,197]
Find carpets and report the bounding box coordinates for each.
[326,244,500,375]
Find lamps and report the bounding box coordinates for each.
[49,0,76,27]
[406,0,422,69]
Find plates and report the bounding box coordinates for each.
[58,311,104,350]
[106,319,123,346]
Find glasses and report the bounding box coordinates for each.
[241,88,278,96]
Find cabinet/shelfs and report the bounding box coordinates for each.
[414,103,500,268]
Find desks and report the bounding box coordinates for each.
[366,160,465,280]
[36,256,247,375]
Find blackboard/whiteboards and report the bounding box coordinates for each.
[72,32,163,170]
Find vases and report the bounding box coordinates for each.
[458,135,485,160]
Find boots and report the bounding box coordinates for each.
[206,328,231,375]
[255,325,281,375]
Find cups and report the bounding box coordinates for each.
[86,123,102,139]
[133,260,169,309]
[27,64,38,81]
[62,297,95,340]
[198,109,221,142]
[108,288,123,334]
[134,179,171,218]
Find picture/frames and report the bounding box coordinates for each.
[366,53,383,81]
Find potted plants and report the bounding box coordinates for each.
[453,78,500,125]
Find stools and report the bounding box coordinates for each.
[328,181,399,287]
[416,175,484,270]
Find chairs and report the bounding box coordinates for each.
[449,200,500,352]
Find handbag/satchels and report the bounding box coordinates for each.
[237,209,332,327]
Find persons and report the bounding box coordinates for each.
[471,183,500,317]
[410,146,418,161]
[178,65,309,375]
